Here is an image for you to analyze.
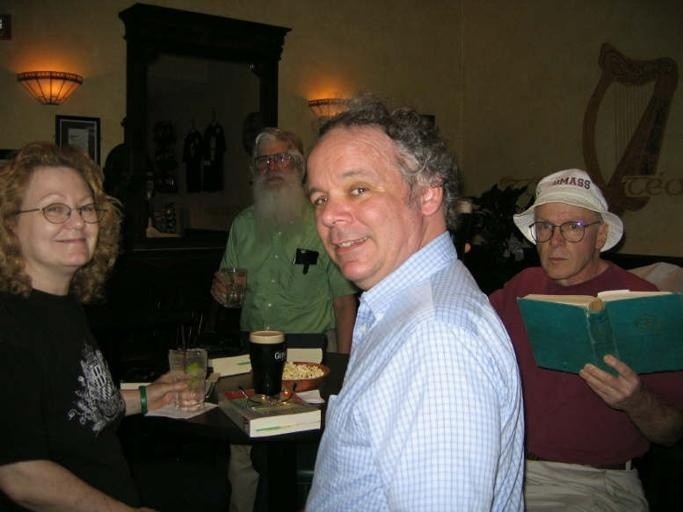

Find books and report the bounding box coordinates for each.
[515,288,679,376]
[207,332,329,378]
[217,389,322,439]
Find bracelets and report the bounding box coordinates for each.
[138,387,149,413]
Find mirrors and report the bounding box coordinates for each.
[119,3,291,252]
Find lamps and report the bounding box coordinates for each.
[17,70,84,105]
[308,97,357,120]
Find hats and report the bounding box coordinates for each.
[513,169,624,252]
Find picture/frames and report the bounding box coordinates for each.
[55,114,101,166]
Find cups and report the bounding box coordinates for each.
[221,268,247,308]
[249,330,287,397]
[168,348,208,412]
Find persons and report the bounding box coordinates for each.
[0,141,206,512]
[488,167,679,512]
[210,126,361,509]
[305,93,526,512]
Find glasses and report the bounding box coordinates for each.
[7,201,108,225]
[252,152,295,170]
[234,384,298,410]
[529,221,601,244]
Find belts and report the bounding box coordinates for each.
[526,458,635,471]
